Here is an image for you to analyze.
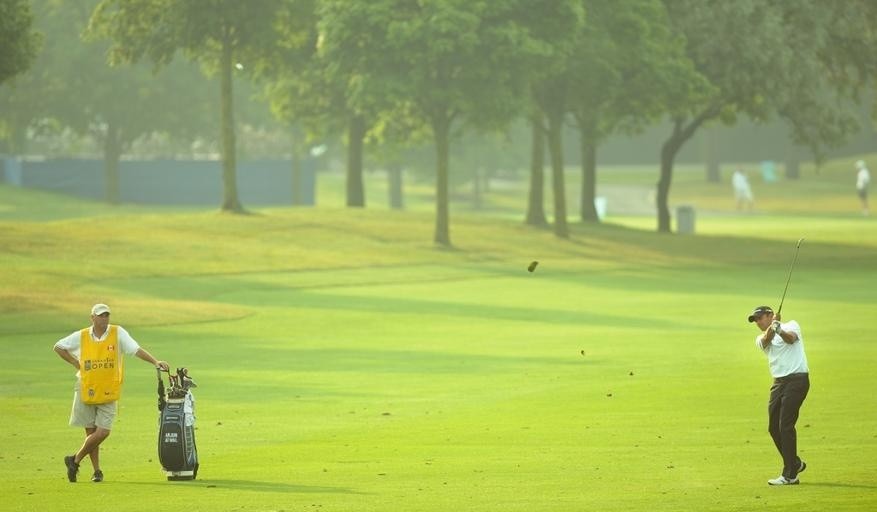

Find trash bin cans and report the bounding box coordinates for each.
[676,206,695,232]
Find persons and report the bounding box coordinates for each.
[731,165,753,212]
[746,307,809,483]
[53,304,169,482]
[853,158,872,210]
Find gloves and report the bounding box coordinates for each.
[770,320,781,335]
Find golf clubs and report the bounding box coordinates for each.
[187,426,197,466]
[774,239,802,333]
[166,367,196,398]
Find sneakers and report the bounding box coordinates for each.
[767,462,806,485]
[92,470,103,481]
[64,456,80,482]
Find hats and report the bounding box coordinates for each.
[92,304,110,316]
[748,306,773,322]
